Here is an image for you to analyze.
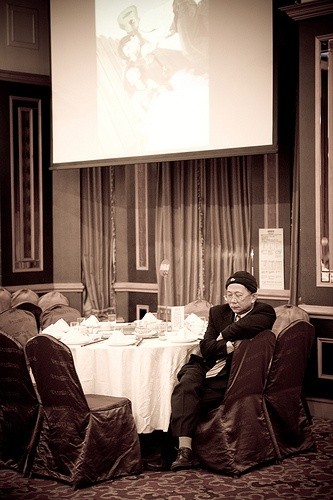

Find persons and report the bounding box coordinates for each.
[145,270,277,472]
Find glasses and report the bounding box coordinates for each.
[223,293,251,302]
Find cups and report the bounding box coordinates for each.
[76,318,85,327]
[70,322,79,332]
[158,323,167,342]
[107,313,117,331]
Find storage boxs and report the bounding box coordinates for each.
[158,303,184,338]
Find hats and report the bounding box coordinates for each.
[226,270,257,293]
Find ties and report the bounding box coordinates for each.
[205,317,240,378]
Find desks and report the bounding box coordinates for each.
[30,320,206,470]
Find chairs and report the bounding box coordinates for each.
[0,287,141,489]
[184,301,317,477]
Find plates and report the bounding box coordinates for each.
[169,337,199,342]
[109,339,137,346]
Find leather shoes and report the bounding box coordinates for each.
[146,454,174,470]
[170,448,200,469]
